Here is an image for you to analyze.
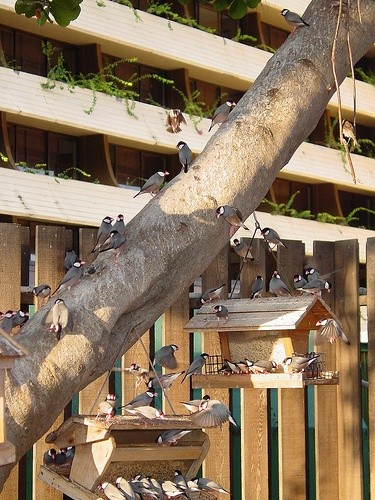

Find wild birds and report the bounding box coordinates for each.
[38,297,71,342]
[279,7,310,33]
[41,444,76,468]
[292,267,333,297]
[213,204,250,232]
[199,283,227,305]
[165,108,188,135]
[260,226,289,252]
[281,353,323,379]
[154,428,193,447]
[0,309,29,335]
[117,344,185,428]
[179,394,239,428]
[315,317,352,346]
[93,392,119,423]
[96,469,233,500]
[268,270,292,298]
[213,304,230,324]
[132,167,170,199]
[51,247,86,297]
[232,238,256,264]
[90,213,127,254]
[249,274,265,301]
[176,140,194,174]
[31,282,53,307]
[208,99,237,133]
[337,116,361,149]
[180,353,210,384]
[216,357,279,375]
